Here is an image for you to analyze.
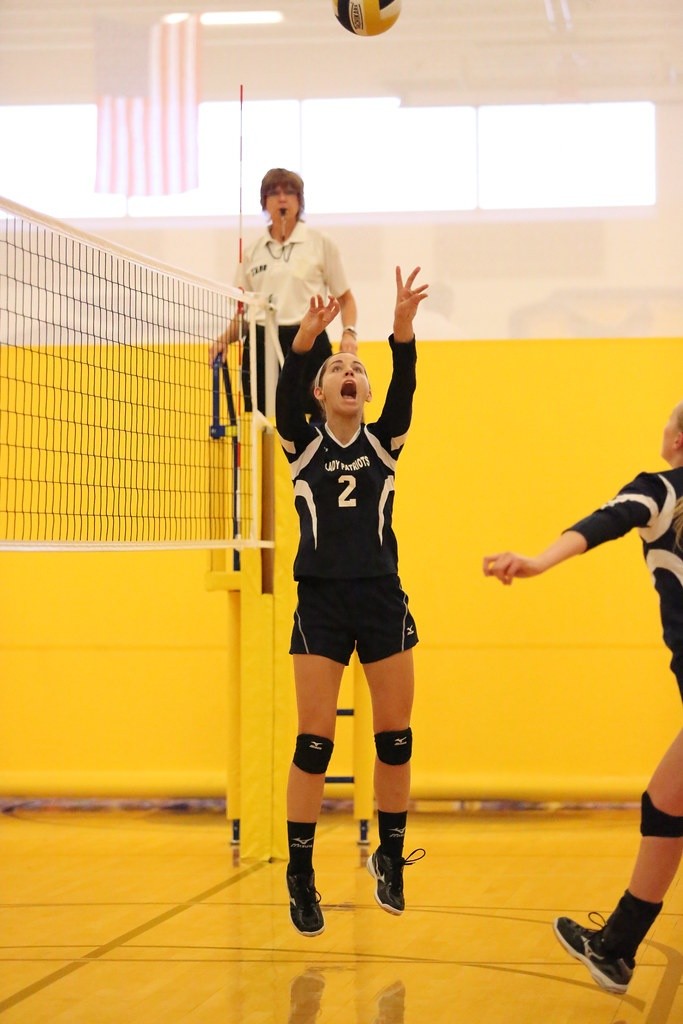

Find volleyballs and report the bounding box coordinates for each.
[330,0,404,37]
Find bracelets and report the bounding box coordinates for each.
[342,326,358,341]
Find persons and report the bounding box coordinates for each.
[482,402,683,994]
[273,266,429,939]
[209,166,359,570]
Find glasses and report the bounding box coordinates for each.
[266,189,299,197]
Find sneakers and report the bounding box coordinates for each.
[366,849,406,917]
[284,859,326,937]
[550,916,636,994]
